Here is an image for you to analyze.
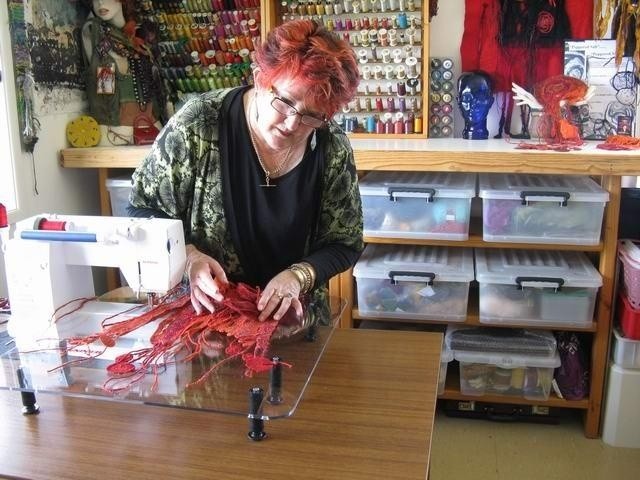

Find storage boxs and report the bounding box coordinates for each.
[601,296,640,450]
[105,178,133,216]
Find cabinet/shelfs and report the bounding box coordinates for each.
[265,0,430,139]
[119,0,265,121]
[340,137,640,439]
[59,143,340,326]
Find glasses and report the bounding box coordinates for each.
[271,86,327,128]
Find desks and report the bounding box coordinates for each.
[0,327,445,480]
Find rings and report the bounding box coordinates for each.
[273,292,285,299]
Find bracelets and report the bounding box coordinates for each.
[288,260,316,294]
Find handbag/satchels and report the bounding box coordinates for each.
[134,114,160,145]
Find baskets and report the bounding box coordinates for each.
[617,238,640,311]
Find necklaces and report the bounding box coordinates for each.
[100,21,149,112]
[247,91,295,188]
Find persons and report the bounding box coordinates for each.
[511,81,596,110]
[454,70,494,140]
[79,0,167,127]
[125,18,365,327]
